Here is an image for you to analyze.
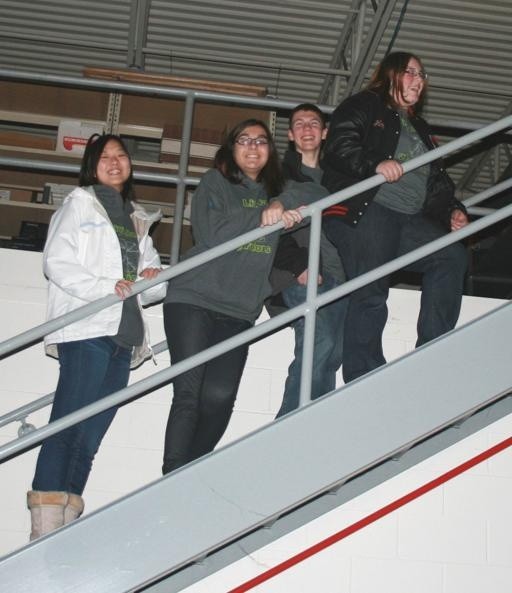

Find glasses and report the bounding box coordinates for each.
[236,138,268,145]
[405,68,428,79]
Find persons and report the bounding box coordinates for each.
[322,47,474,385]
[265,100,349,422]
[21,131,166,543]
[159,114,329,478]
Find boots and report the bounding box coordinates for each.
[27,491,84,541]
